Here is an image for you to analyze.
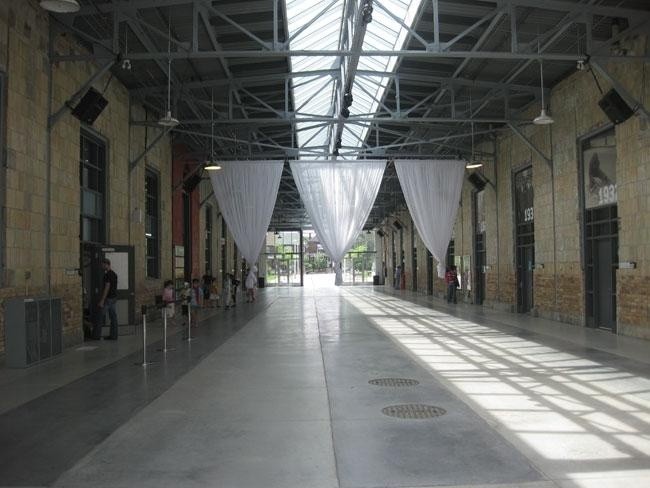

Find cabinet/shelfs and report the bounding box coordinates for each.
[6,295,64,368]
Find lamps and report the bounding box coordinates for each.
[39,0,222,170]
[465,42,554,169]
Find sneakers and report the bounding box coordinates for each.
[89,335,100,340]
[104,335,118,340]
[247,300,253,303]
[212,305,236,310]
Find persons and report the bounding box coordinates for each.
[87,256,119,341]
[446,266,458,304]
[394,266,402,290]
[444,264,452,299]
[160,280,177,327]
[178,257,260,326]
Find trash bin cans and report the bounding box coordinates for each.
[259,277,264,288]
[374,276,379,285]
